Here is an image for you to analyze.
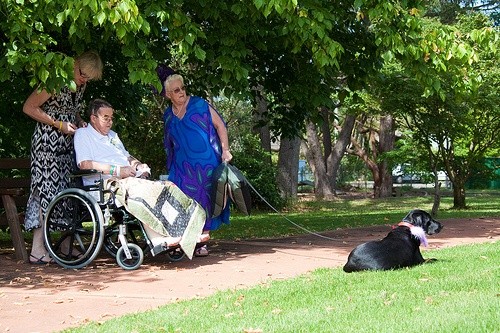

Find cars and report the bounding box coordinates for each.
[392,163,433,184]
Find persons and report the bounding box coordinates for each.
[74,99,211,246]
[21,51,103,264]
[162,73,234,257]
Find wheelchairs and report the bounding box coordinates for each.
[41,149,209,269]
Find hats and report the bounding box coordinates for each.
[153,64,180,95]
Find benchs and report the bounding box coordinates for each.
[0,158,35,263]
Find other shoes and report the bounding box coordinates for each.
[195,246,209,256]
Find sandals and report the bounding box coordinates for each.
[29,252,58,264]
[58,251,82,259]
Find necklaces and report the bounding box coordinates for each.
[173,105,182,116]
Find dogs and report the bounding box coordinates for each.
[343,208,444,273]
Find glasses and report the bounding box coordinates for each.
[79,67,87,78]
[167,86,187,94]
[93,115,115,122]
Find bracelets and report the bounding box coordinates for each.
[135,163,139,171]
[51,119,56,126]
[58,121,62,130]
[116,165,120,177]
[110,164,115,176]
